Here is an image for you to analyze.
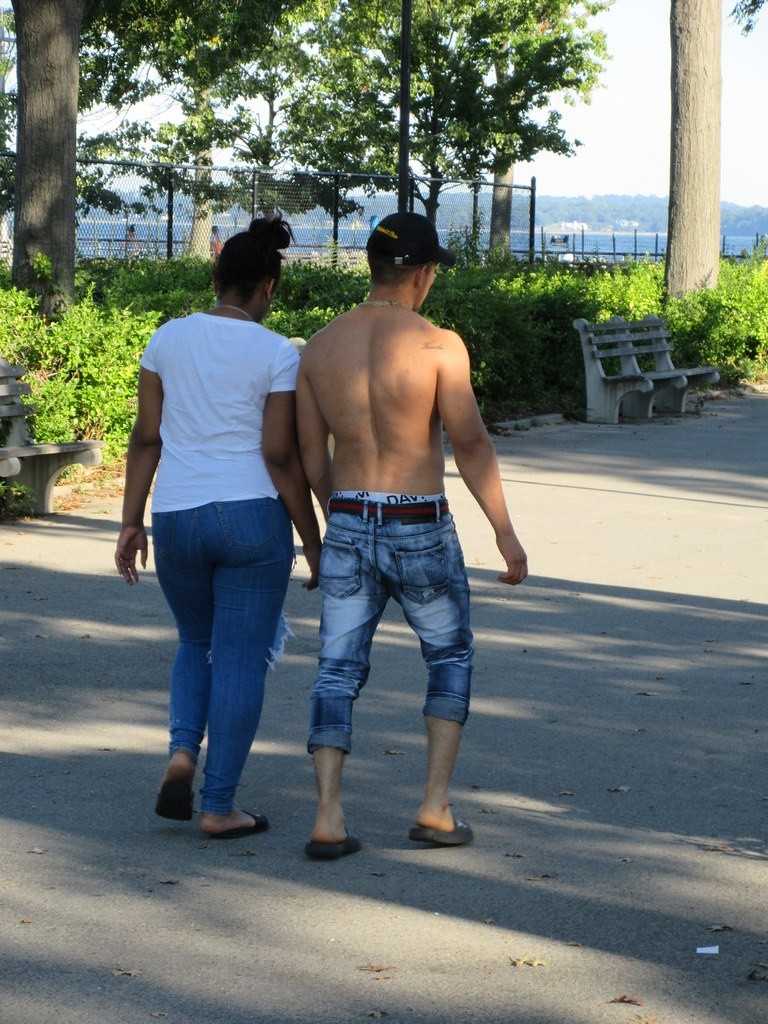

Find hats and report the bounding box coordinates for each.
[365,212,457,268]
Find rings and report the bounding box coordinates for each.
[120,558,125,561]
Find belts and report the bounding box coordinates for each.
[328,498,449,521]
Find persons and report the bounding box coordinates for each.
[120,224,141,261]
[295,213,528,858]
[210,225,221,253]
[114,217,321,839]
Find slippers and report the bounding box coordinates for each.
[305,827,361,858]
[155,780,194,821]
[210,811,268,839]
[406,819,471,844]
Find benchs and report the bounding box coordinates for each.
[572,313,725,424]
[0,357,104,515]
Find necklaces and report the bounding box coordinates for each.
[359,299,400,307]
[213,304,254,321]
